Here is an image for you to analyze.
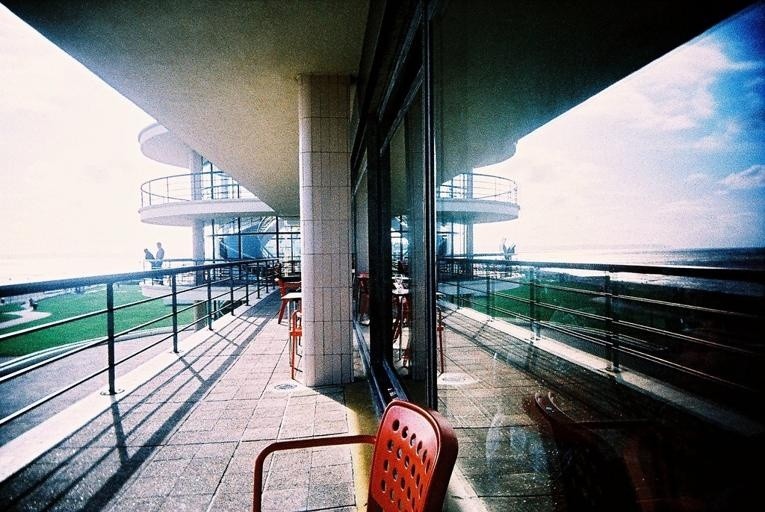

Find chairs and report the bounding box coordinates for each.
[274,276,302,324]
[522,391,706,511]
[252,396,458,512]
[354,269,447,373]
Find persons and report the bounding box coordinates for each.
[155,242,165,285]
[143,248,155,270]
